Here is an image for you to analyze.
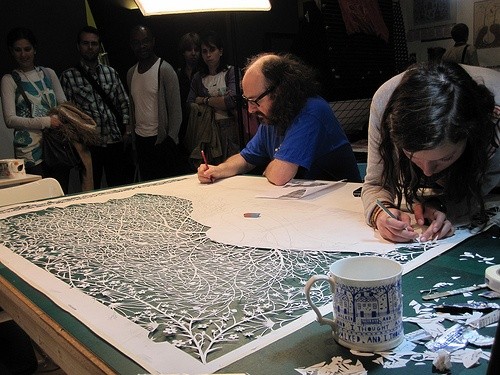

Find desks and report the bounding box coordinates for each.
[0,174,500,375]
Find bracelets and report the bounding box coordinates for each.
[201,96,210,105]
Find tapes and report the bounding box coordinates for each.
[485,264,500,292]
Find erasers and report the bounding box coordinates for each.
[244,212,260,218]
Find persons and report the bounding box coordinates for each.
[175,32,203,103]
[441,23,479,67]
[1,33,68,195]
[186,30,244,172]
[197,52,363,186]
[360,61,500,243]
[61,26,128,191]
[126,24,182,179]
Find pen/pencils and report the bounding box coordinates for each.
[376,199,419,243]
[201,151,214,183]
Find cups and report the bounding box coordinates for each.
[305,256,404,351]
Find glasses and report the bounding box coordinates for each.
[242,81,280,107]
